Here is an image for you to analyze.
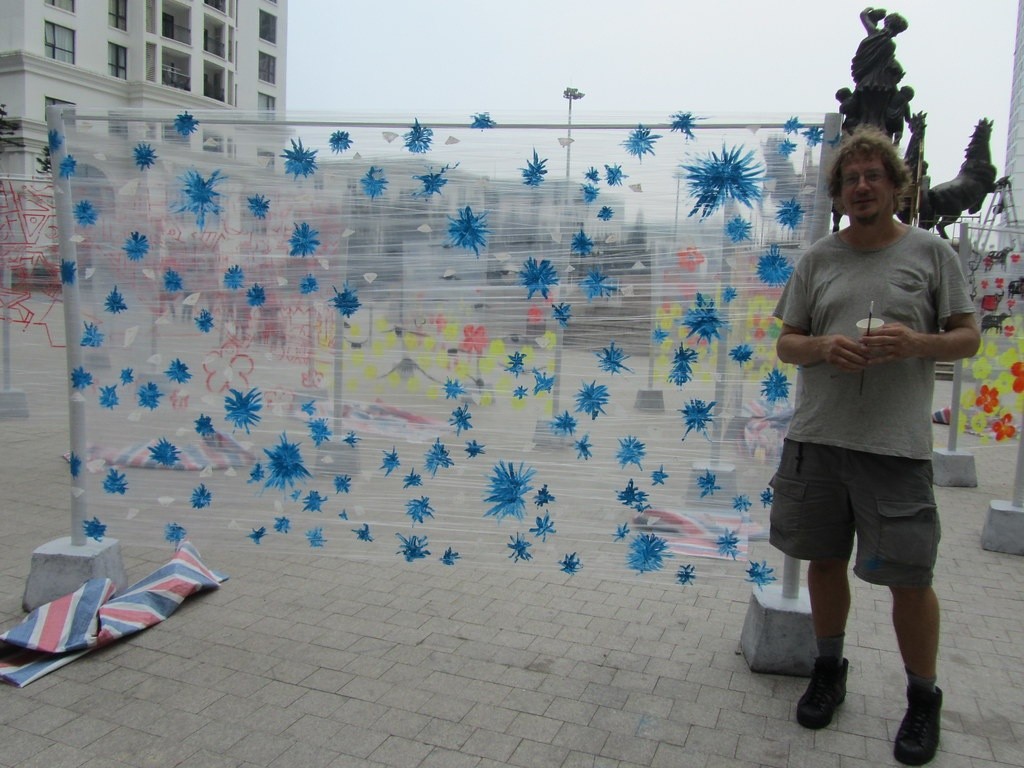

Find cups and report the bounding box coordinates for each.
[856,318,885,362]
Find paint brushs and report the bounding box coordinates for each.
[857,299,875,397]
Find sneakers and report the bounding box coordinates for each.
[893,685,943,766]
[797,656,849,729]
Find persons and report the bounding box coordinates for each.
[768,124,982,763]
[834,7,914,149]
